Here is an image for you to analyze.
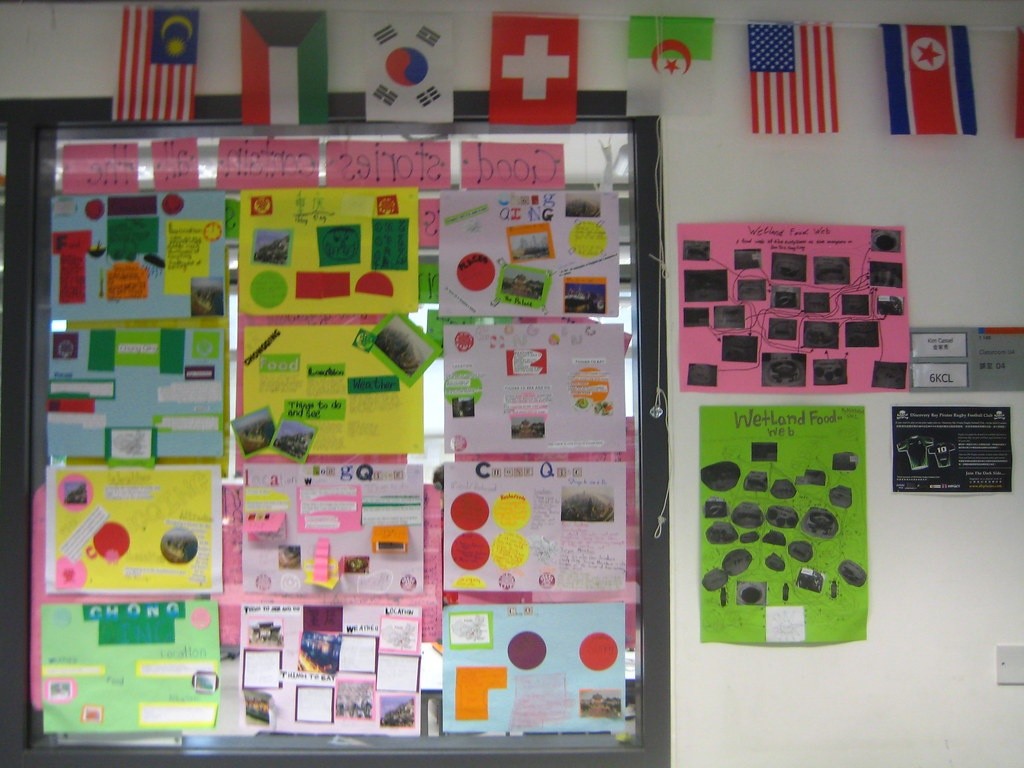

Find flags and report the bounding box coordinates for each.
[878,23,977,136]
[746,18,841,134]
[239,7,329,125]
[1015,25,1024,139]
[361,10,454,123]
[625,16,716,133]
[488,12,579,126]
[110,5,200,120]
